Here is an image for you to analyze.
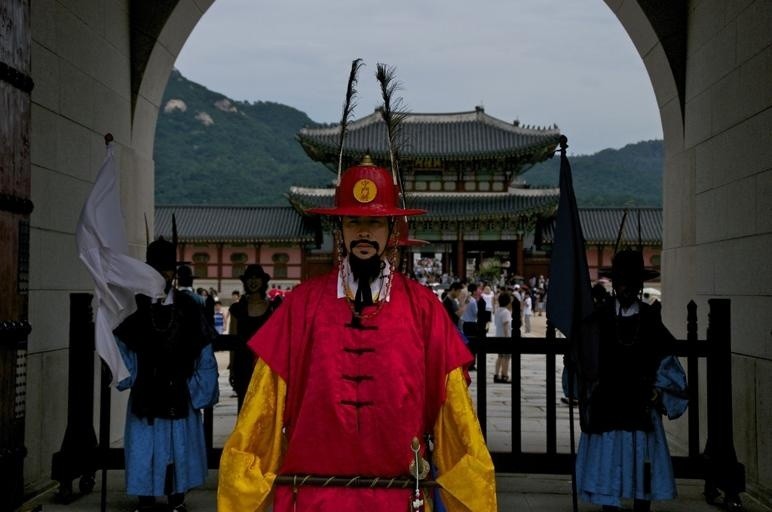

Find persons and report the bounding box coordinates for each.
[554,209,697,510]
[594,278,606,304]
[215,63,503,512]
[192,282,296,340]
[558,355,577,407]
[227,262,269,417]
[401,254,555,385]
[177,262,206,305]
[104,214,225,512]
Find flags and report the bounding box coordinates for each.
[548,155,596,340]
[74,144,166,390]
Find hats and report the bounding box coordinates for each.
[302,162,431,221]
[599,249,664,282]
[236,262,272,281]
[135,234,196,268]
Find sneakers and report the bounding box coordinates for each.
[493,375,511,384]
